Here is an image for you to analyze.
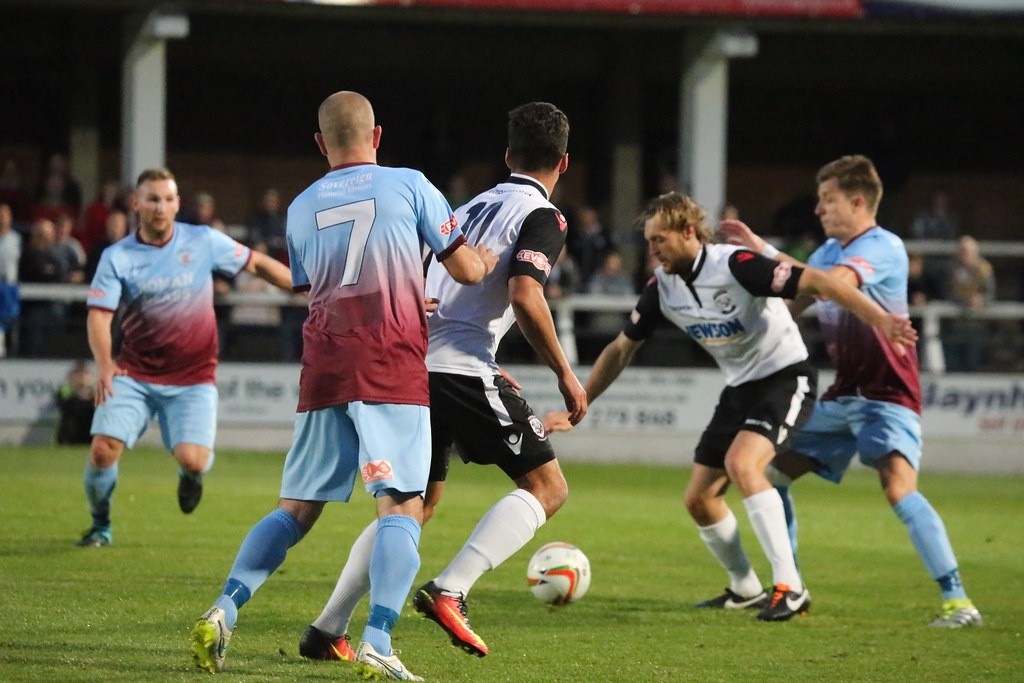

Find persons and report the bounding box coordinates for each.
[545,243,580,298]
[27,162,74,222]
[58,362,95,445]
[586,254,630,364]
[542,191,918,623]
[0,204,88,358]
[572,209,615,272]
[75,169,309,548]
[907,256,944,335]
[85,211,127,288]
[79,181,120,250]
[719,155,982,629]
[189,90,500,681]
[186,192,236,295]
[299,102,588,659]
[947,236,995,370]
[246,186,286,238]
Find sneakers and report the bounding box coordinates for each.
[693,585,770,611]
[76,522,113,548]
[186,604,233,676]
[919,605,983,629]
[412,581,489,659]
[353,641,426,682]
[299,623,357,665]
[178,468,205,515]
[755,581,811,622]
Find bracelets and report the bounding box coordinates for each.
[762,244,781,259]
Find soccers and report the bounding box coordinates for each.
[527,541,591,606]
